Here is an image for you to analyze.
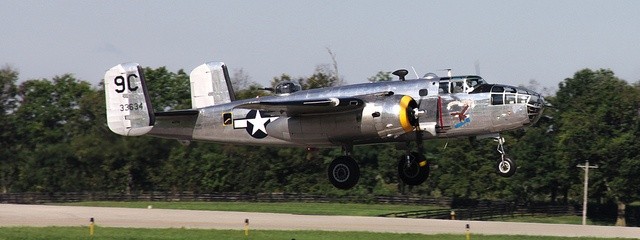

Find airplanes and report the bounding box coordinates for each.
[105,61,558,190]
